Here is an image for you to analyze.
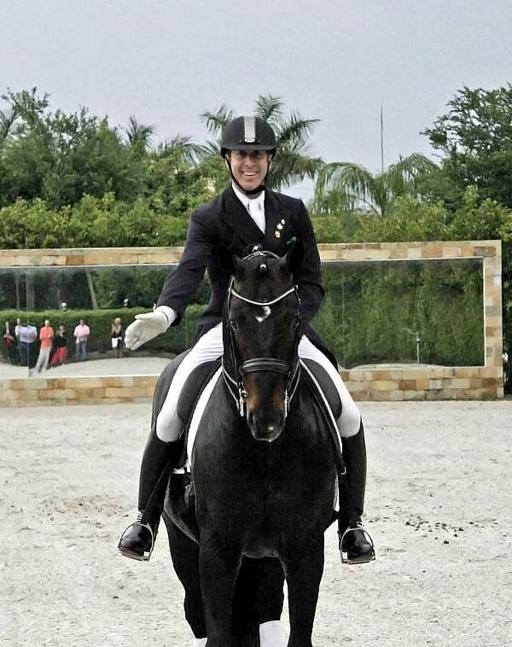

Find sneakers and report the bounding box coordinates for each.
[118,421,184,562]
[10,348,123,377]
[337,417,374,565]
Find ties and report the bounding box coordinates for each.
[247,200,266,234]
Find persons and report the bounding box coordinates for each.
[1,314,124,373]
[116,115,378,565]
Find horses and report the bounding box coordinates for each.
[150,237,346,647]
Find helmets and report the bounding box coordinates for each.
[218,115,278,161]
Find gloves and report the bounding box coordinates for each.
[123,311,169,351]
